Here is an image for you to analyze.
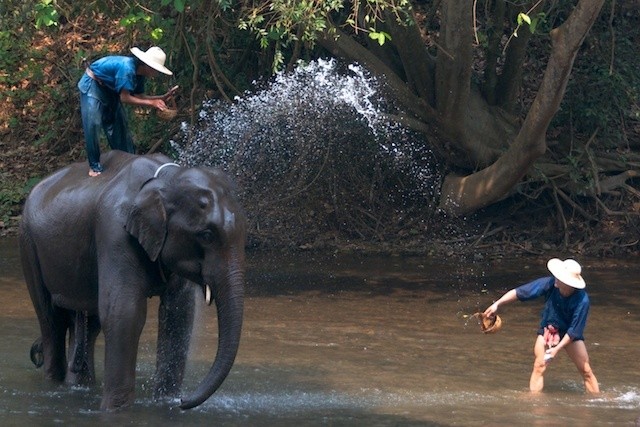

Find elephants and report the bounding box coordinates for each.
[19,148,247,412]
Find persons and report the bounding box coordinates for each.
[78,45,178,177]
[484,258,600,395]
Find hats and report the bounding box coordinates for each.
[547,258,586,289]
[130,46,173,76]
